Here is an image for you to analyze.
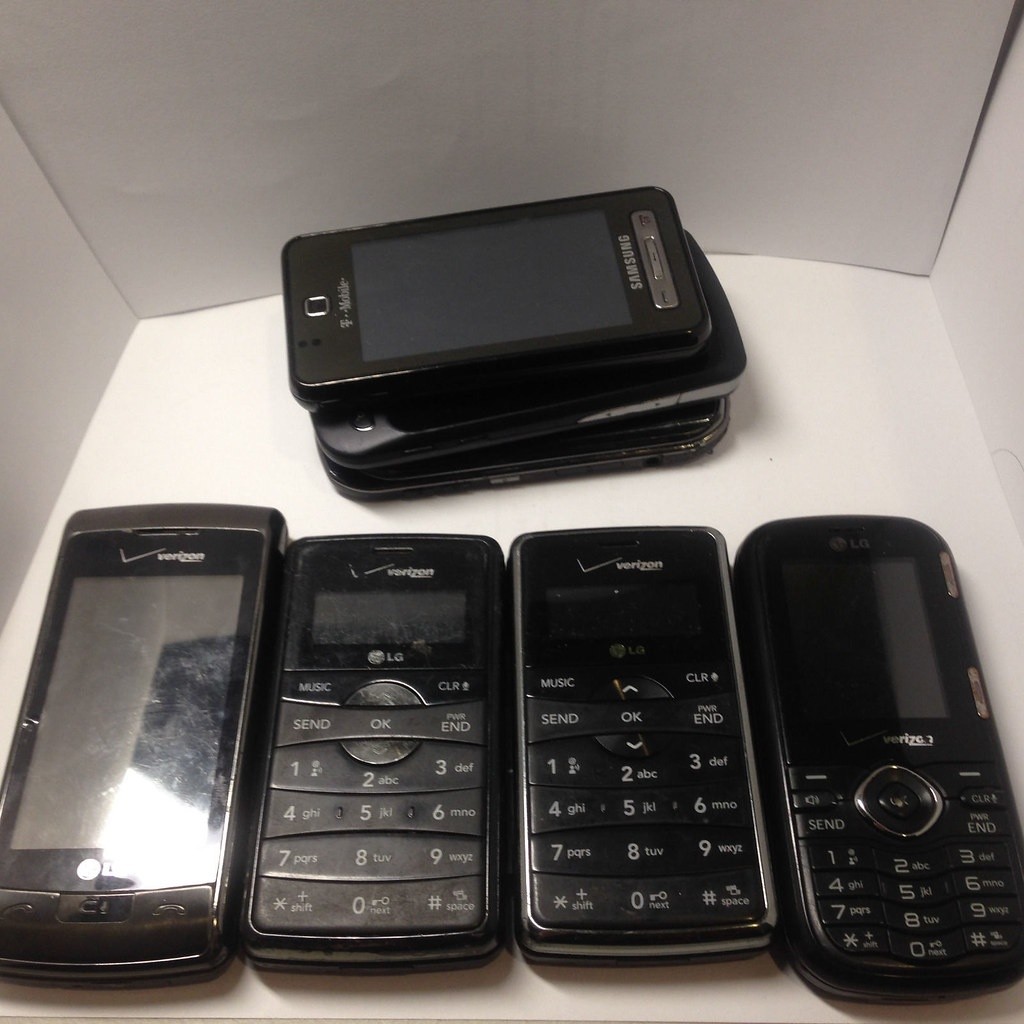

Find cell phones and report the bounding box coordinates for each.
[0,503,295,991]
[233,534,510,978]
[276,186,747,498]
[733,514,1024,1005]
[502,526,781,965]
